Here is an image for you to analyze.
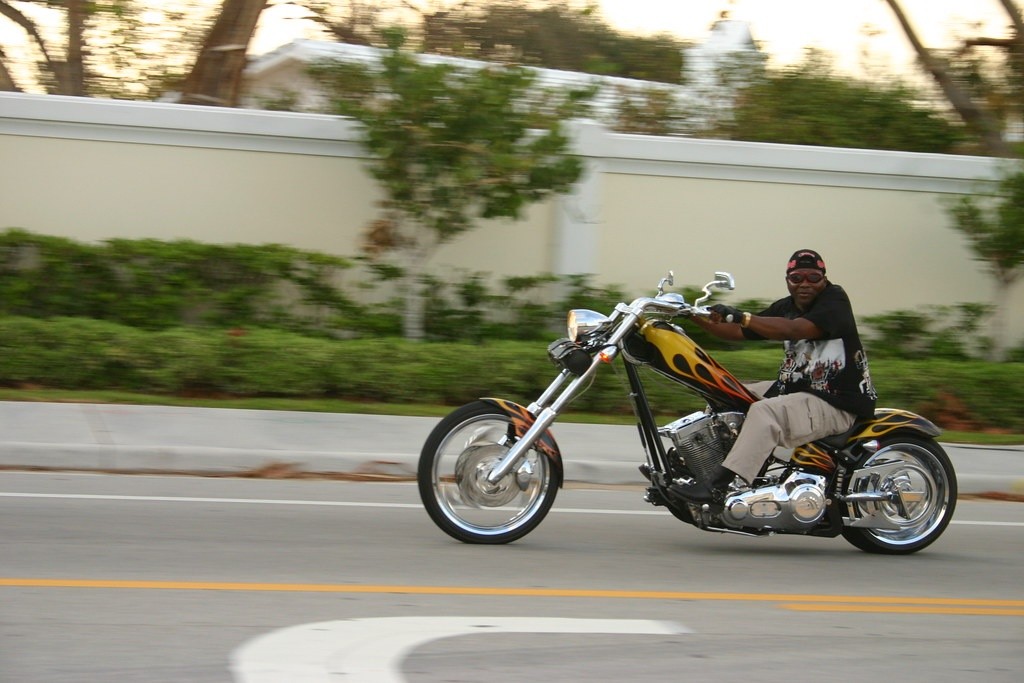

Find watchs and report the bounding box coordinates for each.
[741,312,751,328]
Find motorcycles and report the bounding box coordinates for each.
[417,267,958,545]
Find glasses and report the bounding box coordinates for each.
[788,272,824,284]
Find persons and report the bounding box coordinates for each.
[638,250,875,513]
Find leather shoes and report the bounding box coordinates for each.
[639,463,652,480]
[666,482,725,514]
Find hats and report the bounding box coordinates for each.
[787,250,825,276]
